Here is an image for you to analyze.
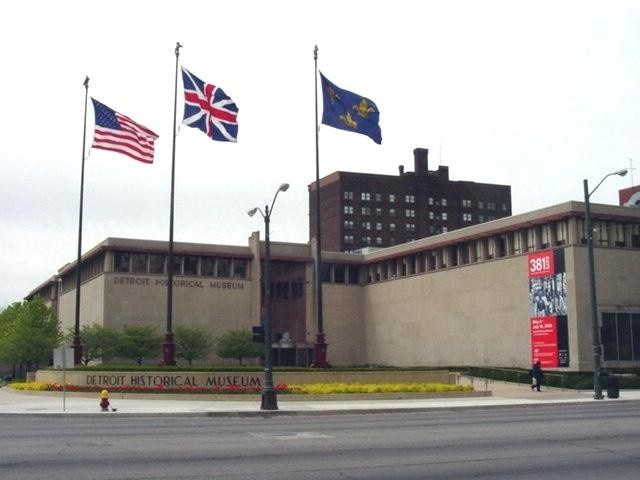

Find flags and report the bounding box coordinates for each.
[181,68,238,144]
[90,98,159,166]
[318,71,382,146]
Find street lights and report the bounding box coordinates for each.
[583,169,628,398]
[249,184,288,410]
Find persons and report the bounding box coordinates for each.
[533,287,566,319]
[529,361,545,391]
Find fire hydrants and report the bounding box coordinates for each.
[98,390,110,412]
